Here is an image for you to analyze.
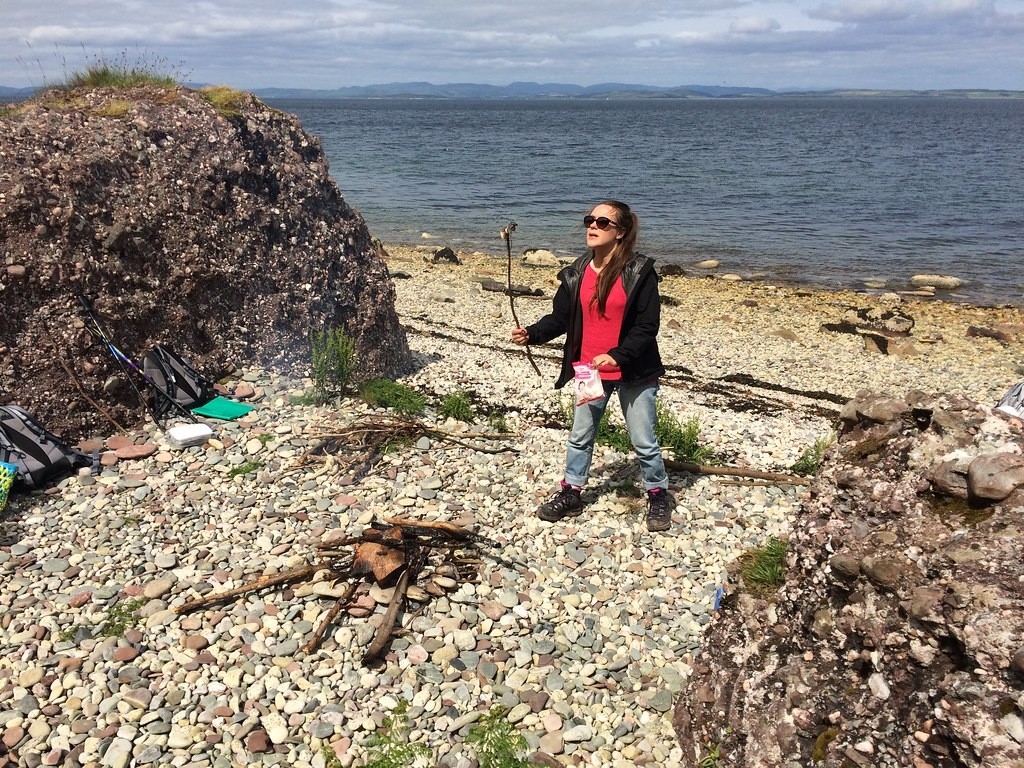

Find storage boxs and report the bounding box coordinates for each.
[169,423,213,449]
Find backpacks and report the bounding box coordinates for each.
[0,404,100,485]
[139,342,214,410]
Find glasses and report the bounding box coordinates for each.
[584,215,620,230]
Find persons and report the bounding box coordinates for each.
[511,199,677,532]
[577,381,596,405]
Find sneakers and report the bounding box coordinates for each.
[646,487,671,530]
[537,480,582,521]
